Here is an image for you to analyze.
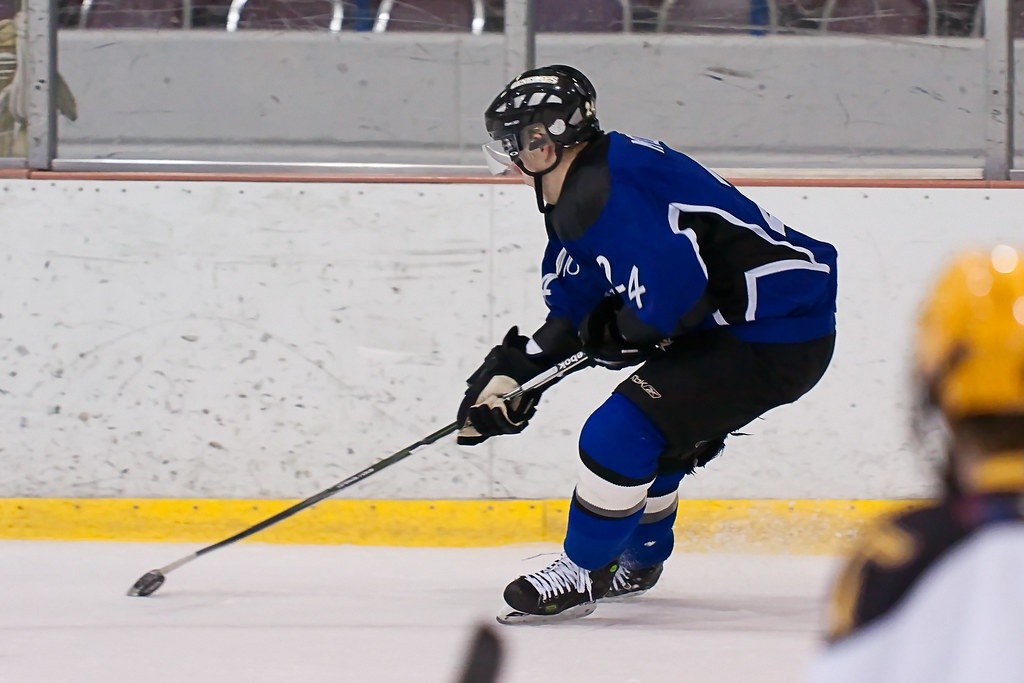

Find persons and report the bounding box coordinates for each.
[451,62,841,616]
[818,252,1024,678]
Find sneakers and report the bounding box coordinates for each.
[496,550,620,625]
[595,550,664,603]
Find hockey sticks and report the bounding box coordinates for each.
[128,348,598,599]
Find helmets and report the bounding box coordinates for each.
[485,65,601,148]
[915,247,1024,414]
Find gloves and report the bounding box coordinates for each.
[457,325,566,446]
[576,295,648,370]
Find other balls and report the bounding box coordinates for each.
[140,576,164,597]
